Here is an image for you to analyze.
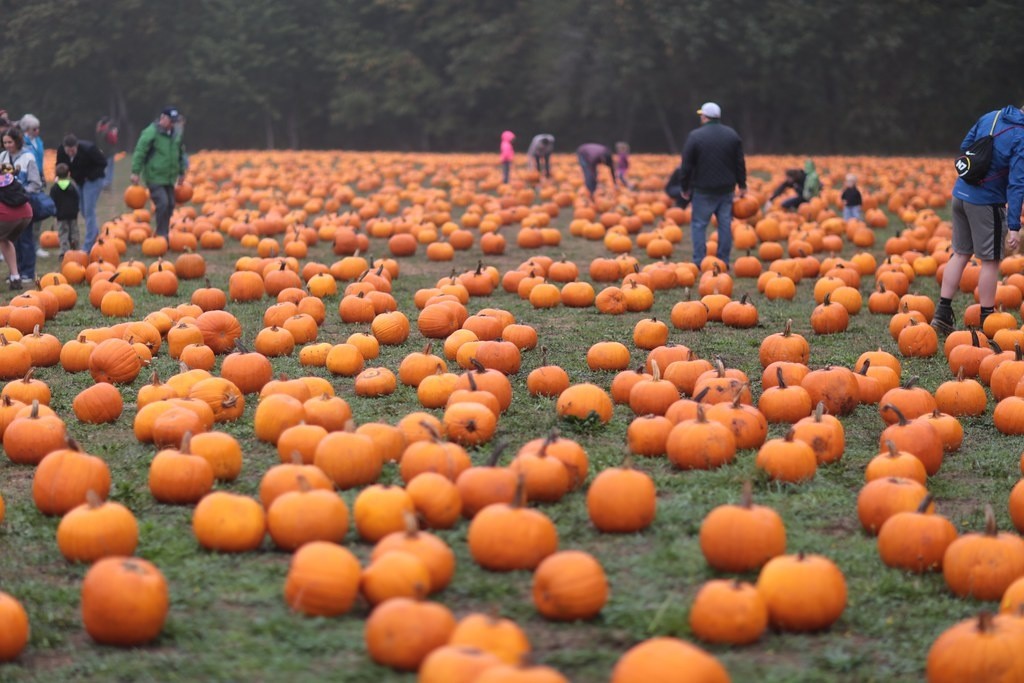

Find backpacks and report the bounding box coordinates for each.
[0,173,27,205]
[955,110,1024,184]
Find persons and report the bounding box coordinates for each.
[0,114,107,290]
[130,109,188,242]
[841,173,862,220]
[500,130,516,183]
[527,133,555,178]
[97,116,118,190]
[665,167,692,209]
[931,105,1024,332]
[577,143,616,193]
[770,159,823,210]
[678,102,749,270]
[615,142,629,184]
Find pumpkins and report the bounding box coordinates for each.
[0,149,1024,683]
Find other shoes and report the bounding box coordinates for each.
[9,279,22,290]
[20,274,33,283]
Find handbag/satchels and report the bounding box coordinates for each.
[28,192,56,221]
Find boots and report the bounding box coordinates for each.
[929,304,956,334]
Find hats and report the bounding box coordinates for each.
[696,102,721,118]
[163,107,179,122]
